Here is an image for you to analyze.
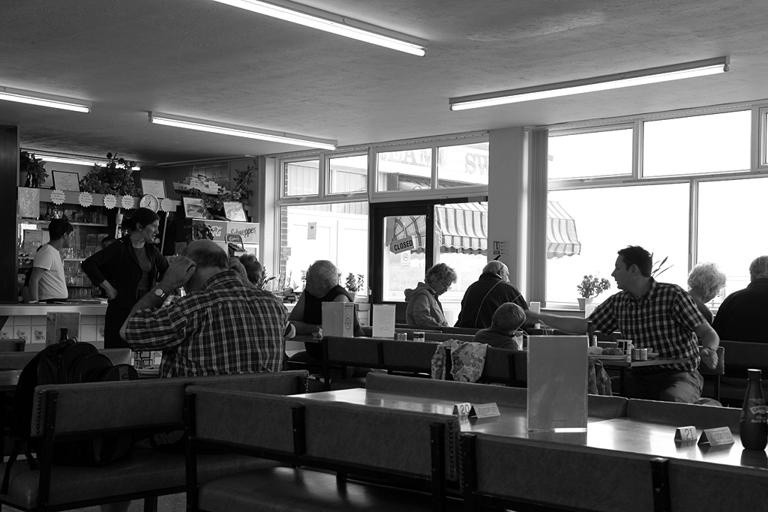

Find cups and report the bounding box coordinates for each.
[616,339,633,363]
[632,348,648,361]
[28,233,109,299]
[412,331,426,343]
[396,332,408,341]
[530,301,541,315]
[512,331,523,351]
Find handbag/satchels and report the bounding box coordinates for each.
[12,339,117,451]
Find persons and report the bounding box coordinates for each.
[22,246,44,304]
[403,262,456,327]
[238,254,262,287]
[288,260,374,392]
[687,263,726,325]
[712,256,768,380]
[454,260,538,330]
[27,218,74,304]
[474,302,526,350]
[80,207,169,349]
[119,238,288,456]
[525,246,720,404]
[91,238,118,298]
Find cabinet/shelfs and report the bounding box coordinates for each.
[17,186,168,299]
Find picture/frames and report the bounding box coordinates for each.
[181,196,206,219]
[52,170,80,192]
[223,200,248,222]
[140,178,167,199]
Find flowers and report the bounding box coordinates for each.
[346,273,365,292]
[577,274,610,299]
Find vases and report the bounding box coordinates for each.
[347,292,356,301]
[578,299,593,309]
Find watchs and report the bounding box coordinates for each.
[150,288,166,300]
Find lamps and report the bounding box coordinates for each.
[214,0,430,57]
[21,149,144,171]
[0,86,93,114]
[149,110,338,151]
[448,56,730,112]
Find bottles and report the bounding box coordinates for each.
[586,321,594,347]
[593,335,597,346]
[739,368,768,451]
